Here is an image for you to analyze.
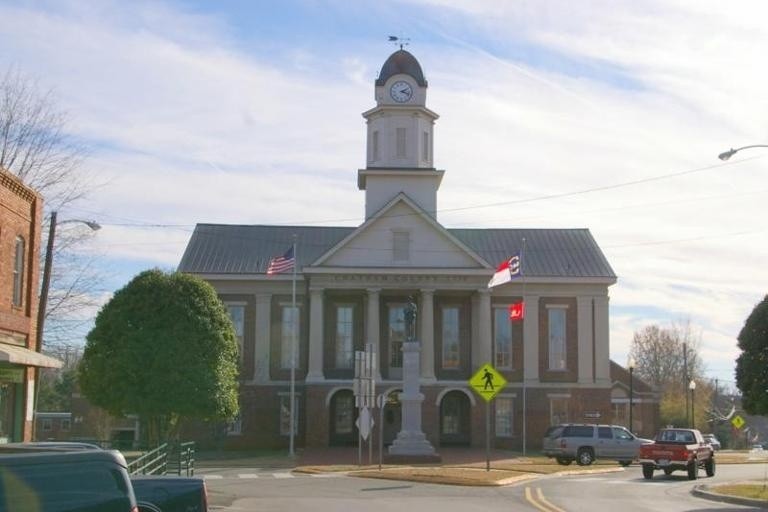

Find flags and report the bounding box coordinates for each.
[266,246,295,277]
[507,303,524,321]
[488,252,522,287]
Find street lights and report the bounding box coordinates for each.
[32,209,105,440]
[687,380,697,429]
[626,355,637,432]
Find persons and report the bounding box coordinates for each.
[403,295,418,342]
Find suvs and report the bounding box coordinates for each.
[541,422,657,468]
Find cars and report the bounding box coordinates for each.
[1,441,209,511]
[752,442,763,451]
[700,432,721,450]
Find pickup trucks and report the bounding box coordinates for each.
[637,424,714,479]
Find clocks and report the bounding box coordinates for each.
[389,80,413,104]
